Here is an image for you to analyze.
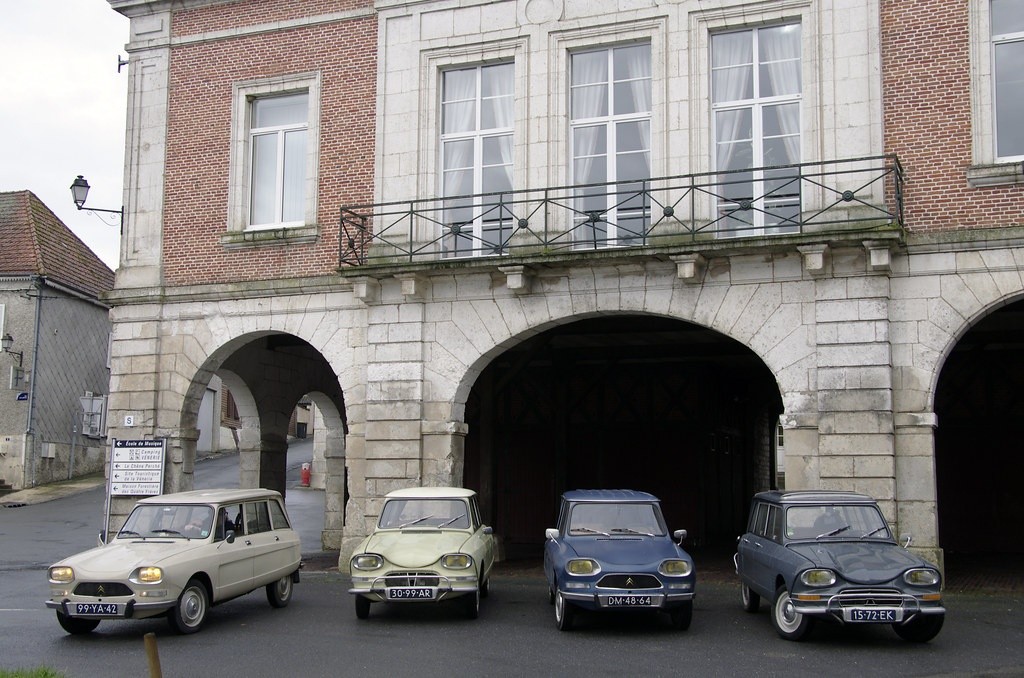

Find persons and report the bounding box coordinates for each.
[182,507,235,539]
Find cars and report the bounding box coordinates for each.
[44,488,307,636]
[348,486,495,620]
[543,488,697,633]
[733,489,946,643]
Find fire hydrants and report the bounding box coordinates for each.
[301,462,310,487]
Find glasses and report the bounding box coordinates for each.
[218,512,228,517]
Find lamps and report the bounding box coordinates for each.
[1,333,23,367]
[70,175,124,235]
